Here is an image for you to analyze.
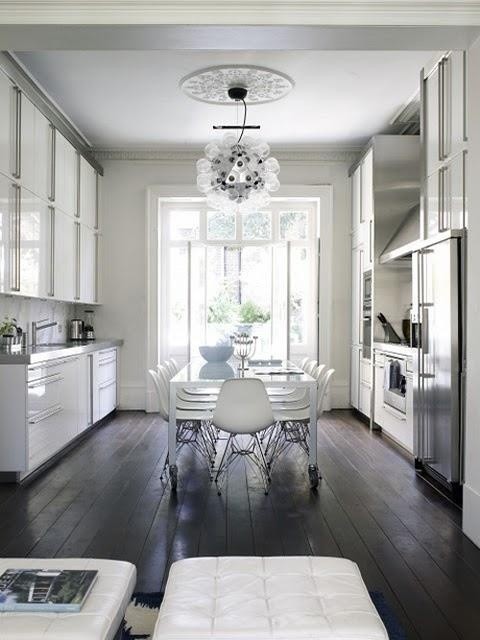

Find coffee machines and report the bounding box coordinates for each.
[83,309,97,340]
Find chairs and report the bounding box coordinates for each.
[150,351,221,484]
[211,376,277,498]
[258,354,337,488]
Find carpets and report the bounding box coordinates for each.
[122,584,407,640]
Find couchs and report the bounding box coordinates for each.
[150,552,392,639]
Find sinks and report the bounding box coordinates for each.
[36,341,70,355]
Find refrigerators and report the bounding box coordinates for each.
[412,227,466,507]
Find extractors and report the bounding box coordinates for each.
[378,203,421,267]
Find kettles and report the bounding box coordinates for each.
[70,318,84,340]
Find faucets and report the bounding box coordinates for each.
[32,318,57,346]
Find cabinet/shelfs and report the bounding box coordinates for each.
[0,69,101,306]
[417,57,469,240]
[348,222,412,432]
[89,346,122,432]
[346,135,418,234]
[0,357,65,486]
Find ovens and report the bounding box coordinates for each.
[362,270,371,359]
[373,350,414,456]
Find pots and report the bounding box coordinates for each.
[402,318,410,342]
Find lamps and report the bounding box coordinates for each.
[192,85,284,221]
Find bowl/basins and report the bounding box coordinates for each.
[198,346,235,363]
[198,363,235,379]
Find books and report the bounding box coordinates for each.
[0,568,99,613]
